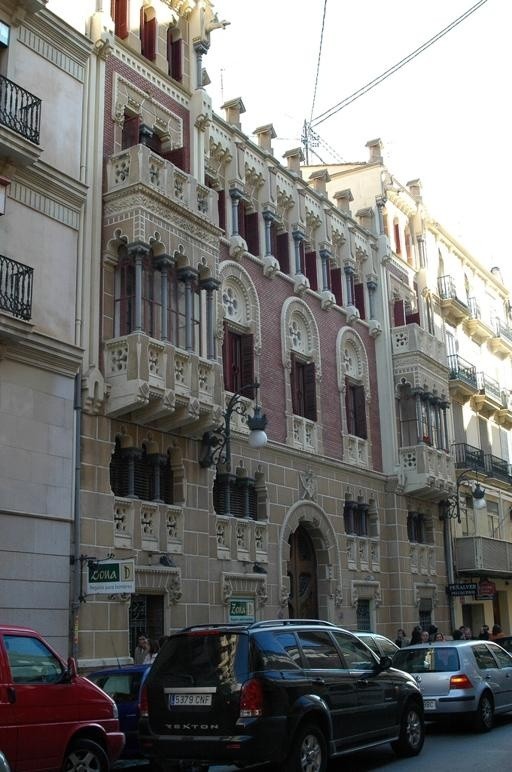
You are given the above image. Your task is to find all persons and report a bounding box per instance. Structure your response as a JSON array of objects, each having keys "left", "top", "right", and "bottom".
[
  {"left": 142, "top": 639, "right": 159, "bottom": 665},
  {"left": 395, "top": 623, "right": 507, "bottom": 649},
  {"left": 133, "top": 630, "right": 151, "bottom": 665}
]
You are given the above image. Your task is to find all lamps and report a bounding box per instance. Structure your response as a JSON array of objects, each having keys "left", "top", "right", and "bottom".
[
  {"left": 199, "top": 376, "right": 267, "bottom": 470},
  {"left": 439, "top": 467, "right": 488, "bottom": 523}
]
